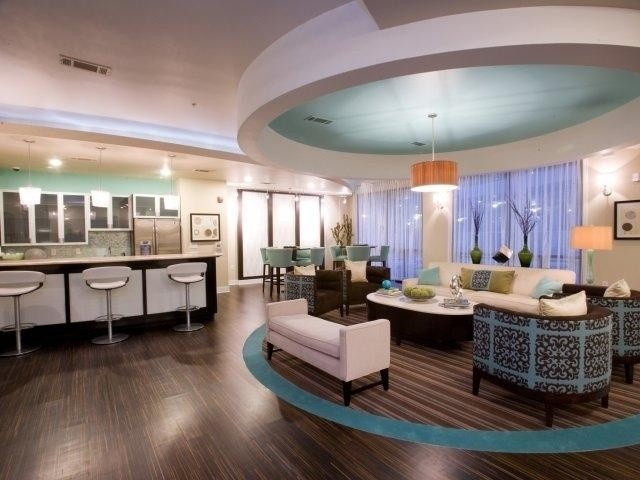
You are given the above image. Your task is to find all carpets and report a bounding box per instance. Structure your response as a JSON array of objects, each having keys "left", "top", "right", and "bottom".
[{"left": 239, "top": 300, "right": 639, "bottom": 455}]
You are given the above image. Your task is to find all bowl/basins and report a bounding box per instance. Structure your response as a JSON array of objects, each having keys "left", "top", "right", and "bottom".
[{"left": 403, "top": 293, "right": 436, "bottom": 303}]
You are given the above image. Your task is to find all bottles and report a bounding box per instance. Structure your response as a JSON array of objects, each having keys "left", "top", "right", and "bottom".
[{"left": 108, "top": 248, "right": 112, "bottom": 257}]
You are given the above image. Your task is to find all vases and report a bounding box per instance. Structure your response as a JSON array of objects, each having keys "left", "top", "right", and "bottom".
[
  {"left": 517, "top": 234, "right": 534, "bottom": 267},
  {"left": 469, "top": 235, "right": 484, "bottom": 265}
]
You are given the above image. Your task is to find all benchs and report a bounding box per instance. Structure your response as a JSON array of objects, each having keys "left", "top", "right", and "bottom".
[{"left": 262, "top": 296, "right": 393, "bottom": 411}]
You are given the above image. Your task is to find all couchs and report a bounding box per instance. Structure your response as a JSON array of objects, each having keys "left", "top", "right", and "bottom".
[{"left": 400, "top": 259, "right": 577, "bottom": 317}]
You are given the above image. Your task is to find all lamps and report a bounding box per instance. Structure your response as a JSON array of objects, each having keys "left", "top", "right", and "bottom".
[
  {"left": 602, "top": 174, "right": 613, "bottom": 197},
  {"left": 163, "top": 154, "right": 182, "bottom": 212},
  {"left": 409, "top": 109, "right": 462, "bottom": 194},
  {"left": 571, "top": 222, "right": 614, "bottom": 286},
  {"left": 89, "top": 143, "right": 111, "bottom": 209},
  {"left": 18, "top": 138, "right": 42, "bottom": 206}
]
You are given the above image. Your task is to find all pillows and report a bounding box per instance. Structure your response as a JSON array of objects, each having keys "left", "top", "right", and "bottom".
[
  {"left": 537, "top": 290, "right": 589, "bottom": 317},
  {"left": 526, "top": 273, "right": 565, "bottom": 301},
  {"left": 601, "top": 275, "right": 632, "bottom": 300},
  {"left": 418, "top": 266, "right": 441, "bottom": 286},
  {"left": 457, "top": 266, "right": 516, "bottom": 294}
]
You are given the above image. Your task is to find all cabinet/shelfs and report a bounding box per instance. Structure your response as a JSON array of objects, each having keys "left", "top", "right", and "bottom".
[
  {"left": 30, "top": 191, "right": 89, "bottom": 247},
  {"left": 86, "top": 193, "right": 134, "bottom": 232},
  {"left": 0, "top": 189, "right": 34, "bottom": 248},
  {"left": 131, "top": 194, "right": 182, "bottom": 219},
  {"left": 128, "top": 217, "right": 181, "bottom": 258}
]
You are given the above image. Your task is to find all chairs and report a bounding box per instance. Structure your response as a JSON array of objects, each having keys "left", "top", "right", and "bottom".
[
  {"left": 164, "top": 261, "right": 211, "bottom": 333},
  {"left": 549, "top": 280, "right": 640, "bottom": 385},
  {"left": 468, "top": 292, "right": 614, "bottom": 427},
  {"left": 257, "top": 241, "right": 393, "bottom": 321},
  {"left": 79, "top": 265, "right": 135, "bottom": 345},
  {"left": 0, "top": 270, "right": 50, "bottom": 360}
]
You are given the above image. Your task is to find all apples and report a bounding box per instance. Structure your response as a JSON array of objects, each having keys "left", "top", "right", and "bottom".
[{"left": 404, "top": 287, "right": 433, "bottom": 296}]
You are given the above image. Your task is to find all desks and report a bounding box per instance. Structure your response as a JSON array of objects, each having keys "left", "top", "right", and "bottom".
[{"left": 0, "top": 249, "right": 224, "bottom": 354}]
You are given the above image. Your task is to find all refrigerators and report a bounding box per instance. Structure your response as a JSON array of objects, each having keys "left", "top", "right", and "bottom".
[{"left": 131, "top": 217, "right": 179, "bottom": 253}]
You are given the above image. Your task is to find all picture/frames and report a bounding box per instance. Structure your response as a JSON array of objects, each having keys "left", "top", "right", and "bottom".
[
  {"left": 612, "top": 199, "right": 640, "bottom": 241},
  {"left": 190, "top": 213, "right": 221, "bottom": 242}
]
